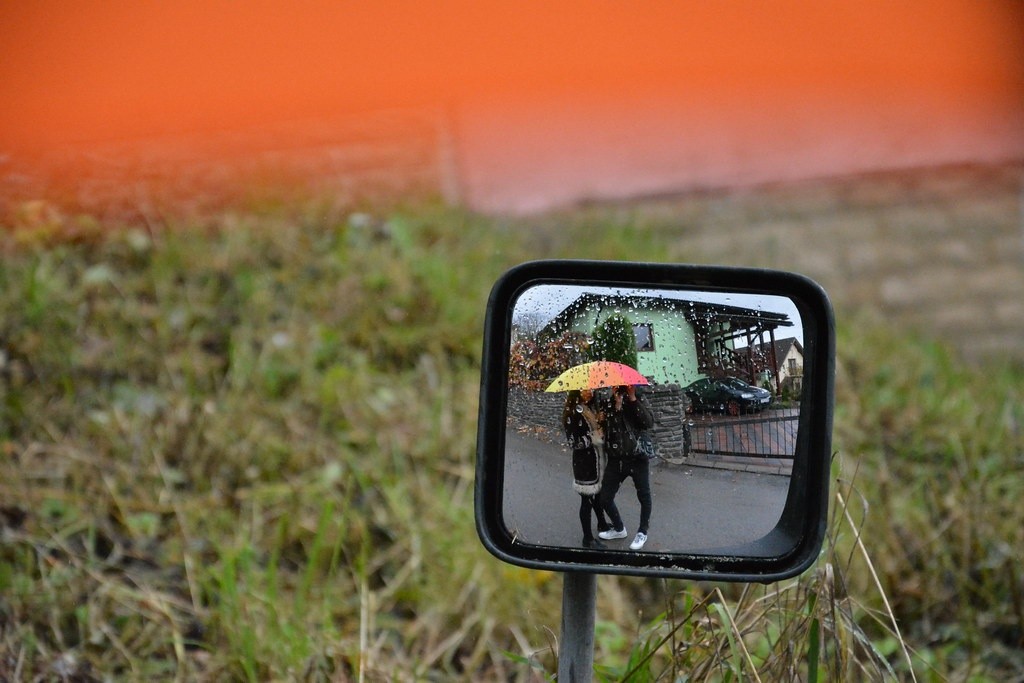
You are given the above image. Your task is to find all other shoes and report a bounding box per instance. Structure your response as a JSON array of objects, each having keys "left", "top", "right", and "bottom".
[
  {"left": 582, "top": 538, "right": 608, "bottom": 549},
  {"left": 597, "top": 523, "right": 614, "bottom": 531}
]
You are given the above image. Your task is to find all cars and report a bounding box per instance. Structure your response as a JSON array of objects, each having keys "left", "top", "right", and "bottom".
[{"left": 682, "top": 376, "right": 772, "bottom": 415}]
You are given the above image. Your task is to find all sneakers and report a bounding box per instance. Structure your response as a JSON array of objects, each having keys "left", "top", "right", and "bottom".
[
  {"left": 599, "top": 527, "right": 628, "bottom": 539},
  {"left": 629, "top": 533, "right": 648, "bottom": 549}
]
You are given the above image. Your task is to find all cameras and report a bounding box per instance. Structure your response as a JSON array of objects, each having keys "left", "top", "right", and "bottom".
[{"left": 616, "top": 385, "right": 628, "bottom": 396}]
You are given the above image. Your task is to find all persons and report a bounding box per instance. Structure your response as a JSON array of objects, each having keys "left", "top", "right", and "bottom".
[
  {"left": 598, "top": 385, "right": 654, "bottom": 549},
  {"left": 563, "top": 390, "right": 612, "bottom": 550}
]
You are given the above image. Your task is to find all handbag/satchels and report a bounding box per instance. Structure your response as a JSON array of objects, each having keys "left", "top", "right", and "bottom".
[{"left": 632, "top": 432, "right": 656, "bottom": 458}]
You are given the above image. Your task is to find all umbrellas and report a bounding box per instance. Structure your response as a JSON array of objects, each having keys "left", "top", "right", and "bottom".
[{"left": 545, "top": 361, "right": 647, "bottom": 411}]
